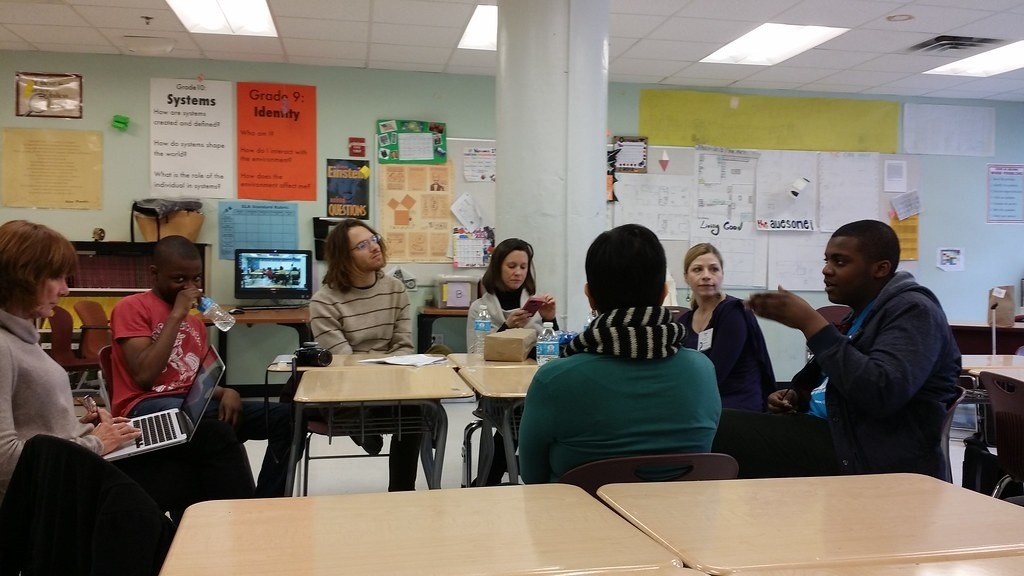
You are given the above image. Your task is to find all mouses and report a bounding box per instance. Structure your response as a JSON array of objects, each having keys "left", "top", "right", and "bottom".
[{"left": 229, "top": 309, "right": 243, "bottom": 314}]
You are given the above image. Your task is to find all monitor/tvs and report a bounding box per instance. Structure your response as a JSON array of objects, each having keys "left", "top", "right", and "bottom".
[{"left": 233, "top": 249, "right": 314, "bottom": 304}]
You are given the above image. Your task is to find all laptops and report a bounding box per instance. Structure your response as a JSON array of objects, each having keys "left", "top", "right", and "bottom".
[{"left": 101, "top": 345, "right": 225, "bottom": 463}]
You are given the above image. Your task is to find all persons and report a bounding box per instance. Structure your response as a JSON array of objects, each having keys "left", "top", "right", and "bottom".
[
  {"left": 308, "top": 218, "right": 425, "bottom": 493},
  {"left": 711, "top": 220, "right": 963, "bottom": 485},
  {"left": 675, "top": 243, "right": 778, "bottom": 414},
  {"left": 111, "top": 235, "right": 308, "bottom": 530},
  {"left": 469, "top": 238, "right": 560, "bottom": 488},
  {"left": 267, "top": 266, "right": 300, "bottom": 283},
  {"left": 0, "top": 220, "right": 143, "bottom": 506},
  {"left": 518, "top": 224, "right": 721, "bottom": 485}
]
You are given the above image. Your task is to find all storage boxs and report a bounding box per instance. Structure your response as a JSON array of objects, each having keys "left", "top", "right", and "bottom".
[{"left": 433, "top": 276, "right": 478, "bottom": 309}]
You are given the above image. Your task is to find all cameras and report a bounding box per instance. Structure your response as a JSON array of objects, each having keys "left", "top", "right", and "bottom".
[{"left": 295, "top": 348, "right": 332, "bottom": 367}]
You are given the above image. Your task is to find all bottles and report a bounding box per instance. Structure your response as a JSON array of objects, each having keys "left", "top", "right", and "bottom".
[
  {"left": 192, "top": 291, "right": 236, "bottom": 332},
  {"left": 583, "top": 309, "right": 594, "bottom": 331},
  {"left": 536, "top": 322, "right": 559, "bottom": 366},
  {"left": 473, "top": 305, "right": 492, "bottom": 360}
]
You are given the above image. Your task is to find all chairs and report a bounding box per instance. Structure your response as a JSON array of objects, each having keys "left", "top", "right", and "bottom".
[
  {"left": 939, "top": 385, "right": 966, "bottom": 484},
  {"left": 74, "top": 300, "right": 111, "bottom": 389},
  {"left": 98, "top": 345, "right": 113, "bottom": 413},
  {"left": 0, "top": 434, "right": 177, "bottom": 576},
  {"left": 559, "top": 453, "right": 739, "bottom": 532},
  {"left": 49, "top": 306, "right": 103, "bottom": 397},
  {"left": 805, "top": 306, "right": 853, "bottom": 363},
  {"left": 980, "top": 371, "right": 1024, "bottom": 499}
]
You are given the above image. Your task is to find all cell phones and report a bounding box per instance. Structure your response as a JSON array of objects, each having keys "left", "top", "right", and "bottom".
[{"left": 522, "top": 297, "right": 544, "bottom": 318}]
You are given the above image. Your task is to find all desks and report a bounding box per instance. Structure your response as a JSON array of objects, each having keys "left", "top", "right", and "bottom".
[{"left": 159, "top": 304, "right": 1024, "bottom": 576}]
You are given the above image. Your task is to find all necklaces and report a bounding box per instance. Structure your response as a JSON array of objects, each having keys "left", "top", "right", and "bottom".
[{"left": 698, "top": 294, "right": 721, "bottom": 333}]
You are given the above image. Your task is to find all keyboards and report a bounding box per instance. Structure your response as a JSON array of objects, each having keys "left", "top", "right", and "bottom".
[{"left": 236, "top": 304, "right": 301, "bottom": 310}]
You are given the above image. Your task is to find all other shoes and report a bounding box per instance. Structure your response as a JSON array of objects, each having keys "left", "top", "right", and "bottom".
[{"left": 350, "top": 432, "right": 382, "bottom": 455}]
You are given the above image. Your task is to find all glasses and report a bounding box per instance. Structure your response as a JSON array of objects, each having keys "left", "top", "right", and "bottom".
[{"left": 349, "top": 233, "right": 381, "bottom": 251}]
